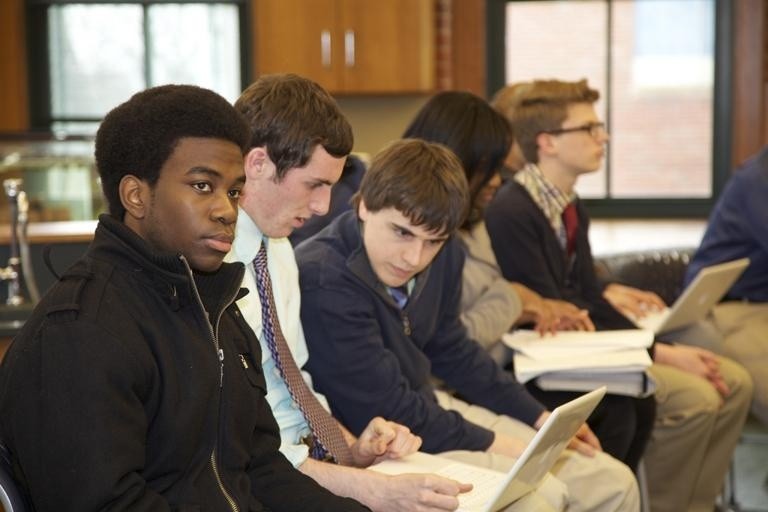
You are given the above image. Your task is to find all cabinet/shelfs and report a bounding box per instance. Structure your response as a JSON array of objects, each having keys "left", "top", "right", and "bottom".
[{"left": 245, "top": 0, "right": 436, "bottom": 98}]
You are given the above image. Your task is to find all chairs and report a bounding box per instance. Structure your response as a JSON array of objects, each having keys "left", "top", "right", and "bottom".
[{"left": 595, "top": 250, "right": 736, "bottom": 510}]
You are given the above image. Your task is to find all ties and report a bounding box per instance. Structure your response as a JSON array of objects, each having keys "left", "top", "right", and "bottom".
[{"left": 253, "top": 241, "right": 355, "bottom": 467}]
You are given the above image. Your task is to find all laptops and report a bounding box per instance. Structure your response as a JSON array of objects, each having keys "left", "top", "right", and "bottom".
[
  {"left": 625, "top": 258, "right": 750, "bottom": 335},
  {"left": 362, "top": 386, "right": 608, "bottom": 510}
]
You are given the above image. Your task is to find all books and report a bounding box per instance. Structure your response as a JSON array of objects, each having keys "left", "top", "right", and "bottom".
[{"left": 501, "top": 326, "right": 659, "bottom": 399}]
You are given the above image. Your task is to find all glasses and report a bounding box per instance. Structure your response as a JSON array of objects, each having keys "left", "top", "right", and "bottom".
[{"left": 547, "top": 122, "right": 605, "bottom": 137}]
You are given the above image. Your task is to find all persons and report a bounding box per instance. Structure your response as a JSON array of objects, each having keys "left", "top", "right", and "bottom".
[
  {"left": 678, "top": 141, "right": 768, "bottom": 496},
  {"left": 402, "top": 88, "right": 656, "bottom": 476},
  {"left": 1, "top": 82, "right": 372, "bottom": 512},
  {"left": 295, "top": 137, "right": 641, "bottom": 512},
  {"left": 489, "top": 83, "right": 534, "bottom": 184},
  {"left": 487, "top": 80, "right": 756, "bottom": 511},
  {"left": 221, "top": 73, "right": 557, "bottom": 512}
]
